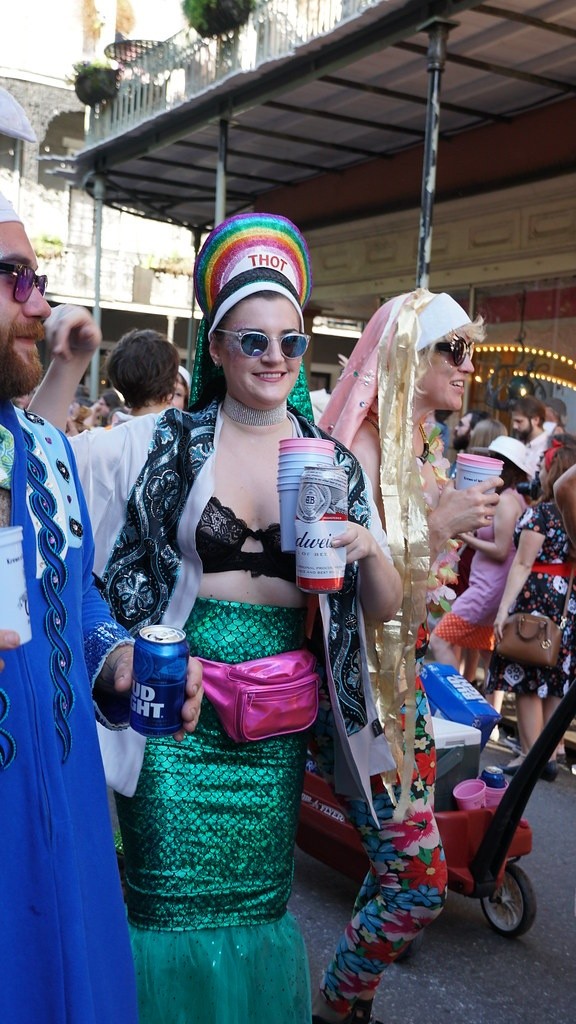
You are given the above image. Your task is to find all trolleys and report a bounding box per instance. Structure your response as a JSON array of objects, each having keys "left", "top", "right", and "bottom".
[{"left": 295, "top": 681, "right": 576, "bottom": 940}]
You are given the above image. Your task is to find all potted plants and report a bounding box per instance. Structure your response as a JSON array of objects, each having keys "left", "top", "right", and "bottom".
[
  {"left": 180, "top": 0, "right": 257, "bottom": 37},
  {"left": 68, "top": 62, "right": 116, "bottom": 106}
]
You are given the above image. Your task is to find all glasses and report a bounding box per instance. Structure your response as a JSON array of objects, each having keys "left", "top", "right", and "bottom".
[
  {"left": 0, "top": 261, "right": 49, "bottom": 304},
  {"left": 424, "top": 334, "right": 476, "bottom": 367},
  {"left": 215, "top": 329, "right": 313, "bottom": 360}
]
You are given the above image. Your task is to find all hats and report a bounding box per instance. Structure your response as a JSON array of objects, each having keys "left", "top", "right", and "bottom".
[
  {"left": 472, "top": 434, "right": 535, "bottom": 479},
  {"left": 0, "top": 191, "right": 24, "bottom": 225}
]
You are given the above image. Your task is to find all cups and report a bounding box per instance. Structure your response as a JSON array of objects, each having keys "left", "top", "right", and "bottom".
[
  {"left": 476, "top": 775, "right": 509, "bottom": 807},
  {"left": 454, "top": 452, "right": 505, "bottom": 519},
  {"left": 276, "top": 437, "right": 337, "bottom": 553},
  {"left": 452, "top": 779, "right": 487, "bottom": 811}
]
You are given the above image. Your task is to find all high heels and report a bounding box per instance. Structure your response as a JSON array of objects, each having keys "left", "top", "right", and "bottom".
[
  {"left": 500, "top": 762, "right": 521, "bottom": 777},
  {"left": 541, "top": 758, "right": 559, "bottom": 782}
]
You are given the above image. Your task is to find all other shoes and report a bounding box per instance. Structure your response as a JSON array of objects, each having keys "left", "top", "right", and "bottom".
[
  {"left": 504, "top": 734, "right": 521, "bottom": 754},
  {"left": 489, "top": 729, "right": 500, "bottom": 742}
]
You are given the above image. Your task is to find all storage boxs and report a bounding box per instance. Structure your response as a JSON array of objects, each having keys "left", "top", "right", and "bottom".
[
  {"left": 418, "top": 662, "right": 501, "bottom": 756},
  {"left": 431, "top": 716, "right": 482, "bottom": 812}
]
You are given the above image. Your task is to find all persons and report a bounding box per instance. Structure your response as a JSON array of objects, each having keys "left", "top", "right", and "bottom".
[
  {"left": 28, "top": 214, "right": 402, "bottom": 1024},
  {"left": 69, "top": 329, "right": 179, "bottom": 577},
  {"left": 11, "top": 365, "right": 193, "bottom": 436},
  {"left": 420, "top": 400, "right": 576, "bottom": 781},
  {"left": 318, "top": 287, "right": 504, "bottom": 1023},
  {"left": 0, "top": 206, "right": 203, "bottom": 1024}
]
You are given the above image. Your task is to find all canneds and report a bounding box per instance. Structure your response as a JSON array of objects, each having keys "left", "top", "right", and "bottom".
[
  {"left": 480, "top": 766, "right": 505, "bottom": 788},
  {"left": 128, "top": 624, "right": 189, "bottom": 737},
  {"left": 295, "top": 465, "right": 349, "bottom": 594}
]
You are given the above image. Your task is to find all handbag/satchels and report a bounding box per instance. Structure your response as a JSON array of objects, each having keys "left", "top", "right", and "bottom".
[
  {"left": 197, "top": 649, "right": 319, "bottom": 743},
  {"left": 496, "top": 611, "right": 562, "bottom": 666}
]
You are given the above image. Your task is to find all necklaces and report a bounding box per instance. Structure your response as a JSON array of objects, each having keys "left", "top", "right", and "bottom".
[
  {"left": 223, "top": 391, "right": 287, "bottom": 425},
  {"left": 416, "top": 422, "right": 429, "bottom": 464}
]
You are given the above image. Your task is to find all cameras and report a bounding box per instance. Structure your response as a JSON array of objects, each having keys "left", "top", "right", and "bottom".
[{"left": 516, "top": 479, "right": 545, "bottom": 500}]
